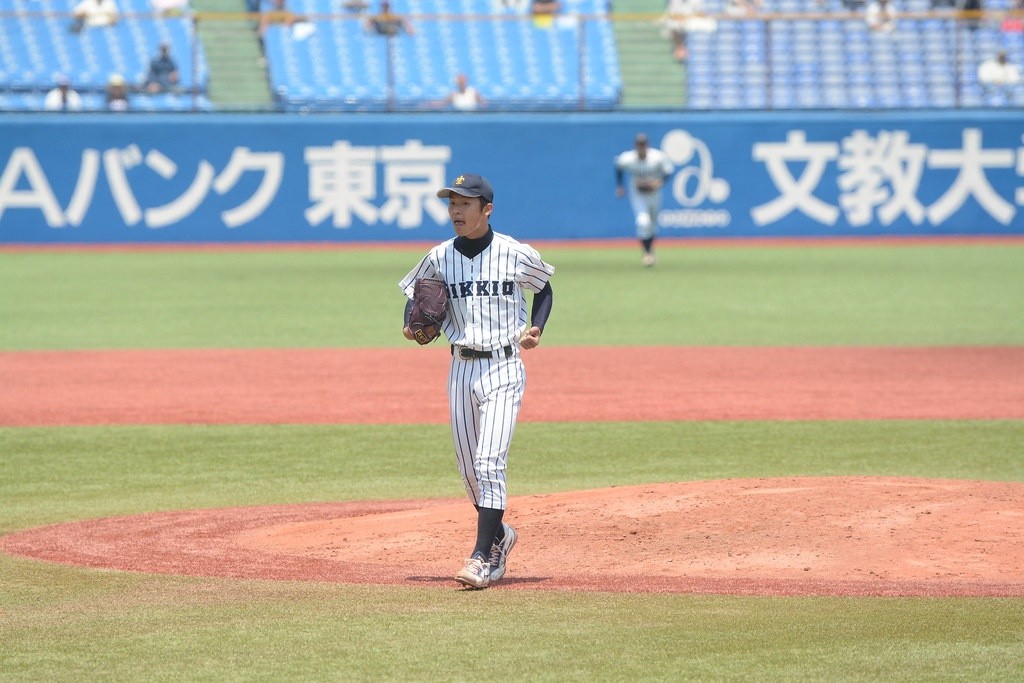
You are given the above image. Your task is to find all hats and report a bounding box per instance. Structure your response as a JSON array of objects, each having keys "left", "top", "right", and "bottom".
[{"left": 437, "top": 174, "right": 493, "bottom": 203}]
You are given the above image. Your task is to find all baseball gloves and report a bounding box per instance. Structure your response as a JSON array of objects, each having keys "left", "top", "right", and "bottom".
[{"left": 408, "top": 276, "right": 449, "bottom": 345}]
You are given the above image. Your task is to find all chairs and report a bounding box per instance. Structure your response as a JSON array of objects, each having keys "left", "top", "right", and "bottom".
[
  {"left": 0, "top": 0, "right": 211, "bottom": 112},
  {"left": 682, "top": 1, "right": 1023, "bottom": 109},
  {"left": 257, "top": 0, "right": 622, "bottom": 111}
]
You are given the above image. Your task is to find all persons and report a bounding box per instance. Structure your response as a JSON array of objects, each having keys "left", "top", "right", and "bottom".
[
  {"left": 429, "top": 71, "right": 484, "bottom": 110},
  {"left": 361, "top": 0, "right": 412, "bottom": 38},
  {"left": 978, "top": 50, "right": 1019, "bottom": 87},
  {"left": 613, "top": 133, "right": 675, "bottom": 264},
  {"left": 399, "top": 175, "right": 557, "bottom": 591},
  {"left": 105, "top": 71, "right": 128, "bottom": 112},
  {"left": 69, "top": 0, "right": 118, "bottom": 31},
  {"left": 866, "top": 2, "right": 896, "bottom": 28},
  {"left": 45, "top": 76, "right": 79, "bottom": 112},
  {"left": 657, "top": 1, "right": 700, "bottom": 61},
  {"left": 141, "top": 44, "right": 178, "bottom": 97},
  {"left": 257, "top": 1, "right": 294, "bottom": 36}
]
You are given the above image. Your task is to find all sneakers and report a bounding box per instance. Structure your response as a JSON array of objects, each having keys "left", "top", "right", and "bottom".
[
  {"left": 454, "top": 558, "right": 490, "bottom": 588},
  {"left": 488, "top": 524, "right": 518, "bottom": 581}
]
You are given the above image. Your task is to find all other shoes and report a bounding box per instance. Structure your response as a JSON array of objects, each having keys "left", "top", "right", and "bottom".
[{"left": 645, "top": 255, "right": 654, "bottom": 263}]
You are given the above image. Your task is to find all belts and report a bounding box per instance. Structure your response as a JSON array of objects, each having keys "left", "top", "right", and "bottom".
[{"left": 451, "top": 345, "right": 513, "bottom": 360}]
